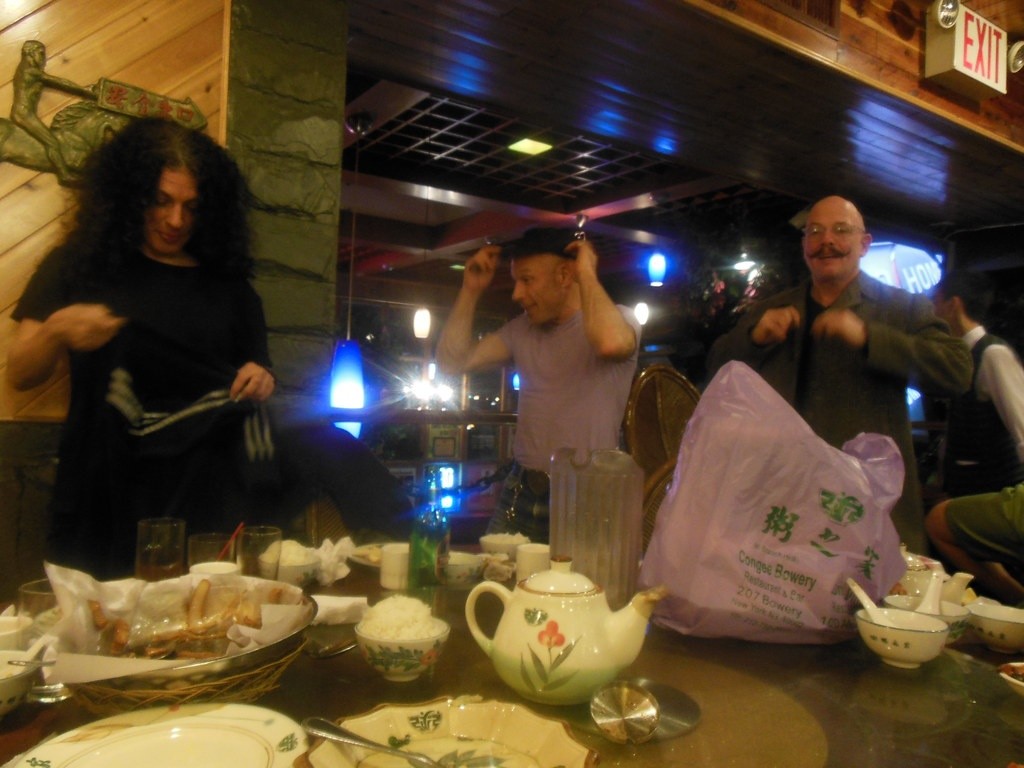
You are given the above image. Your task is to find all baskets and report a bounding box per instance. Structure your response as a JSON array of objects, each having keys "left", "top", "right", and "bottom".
[{"left": 59, "top": 636, "right": 310, "bottom": 717}]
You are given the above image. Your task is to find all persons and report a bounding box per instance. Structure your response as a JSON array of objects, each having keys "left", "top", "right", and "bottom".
[
  {"left": 707, "top": 195, "right": 973, "bottom": 554},
  {"left": 434, "top": 226, "right": 642, "bottom": 544},
  {"left": 6, "top": 117, "right": 278, "bottom": 581},
  {"left": 933, "top": 270, "right": 1024, "bottom": 494},
  {"left": 926, "top": 481, "right": 1024, "bottom": 610}
]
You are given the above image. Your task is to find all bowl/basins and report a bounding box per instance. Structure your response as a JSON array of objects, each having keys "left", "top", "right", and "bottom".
[
  {"left": 449, "top": 561, "right": 485, "bottom": 594},
  {"left": 850, "top": 593, "right": 1022, "bottom": 668},
  {"left": 0, "top": 650, "right": 32, "bottom": 715},
  {"left": 480, "top": 537, "right": 517, "bottom": 562},
  {"left": 258, "top": 556, "right": 321, "bottom": 589},
  {"left": 355, "top": 618, "right": 452, "bottom": 683},
  {"left": 59, "top": 576, "right": 319, "bottom": 718}
]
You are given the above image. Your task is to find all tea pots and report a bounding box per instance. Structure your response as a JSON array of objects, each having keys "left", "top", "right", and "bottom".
[
  {"left": 890, "top": 542, "right": 974, "bottom": 603},
  {"left": 465, "top": 555, "right": 673, "bottom": 705}
]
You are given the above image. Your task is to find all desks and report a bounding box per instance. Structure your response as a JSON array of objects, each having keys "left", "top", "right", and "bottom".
[{"left": 0, "top": 546, "right": 1024, "bottom": 768}]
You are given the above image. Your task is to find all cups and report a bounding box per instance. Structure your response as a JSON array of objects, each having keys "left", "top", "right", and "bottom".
[
  {"left": 0, "top": 614, "right": 33, "bottom": 648},
  {"left": 188, "top": 532, "right": 234, "bottom": 564},
  {"left": 136, "top": 517, "right": 186, "bottom": 579},
  {"left": 237, "top": 527, "right": 282, "bottom": 580},
  {"left": 517, "top": 544, "right": 550, "bottom": 585},
  {"left": 16, "top": 578, "right": 71, "bottom": 702},
  {"left": 188, "top": 562, "right": 237, "bottom": 574},
  {"left": 380, "top": 545, "right": 410, "bottom": 590}
]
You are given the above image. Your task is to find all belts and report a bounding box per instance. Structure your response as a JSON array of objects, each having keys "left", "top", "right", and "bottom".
[{"left": 515, "top": 465, "right": 552, "bottom": 497}]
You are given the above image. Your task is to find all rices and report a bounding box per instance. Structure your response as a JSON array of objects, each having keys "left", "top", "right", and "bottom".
[
  {"left": 357, "top": 595, "right": 442, "bottom": 643},
  {"left": 480, "top": 531, "right": 527, "bottom": 544},
  {"left": 260, "top": 540, "right": 319, "bottom": 567}
]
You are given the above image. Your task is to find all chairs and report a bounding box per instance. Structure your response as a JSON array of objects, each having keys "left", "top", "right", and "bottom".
[
  {"left": 622, "top": 363, "right": 703, "bottom": 464},
  {"left": 640, "top": 457, "right": 676, "bottom": 558}
]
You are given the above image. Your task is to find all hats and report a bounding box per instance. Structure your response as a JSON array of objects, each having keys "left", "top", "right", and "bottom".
[{"left": 494, "top": 226, "right": 577, "bottom": 260}]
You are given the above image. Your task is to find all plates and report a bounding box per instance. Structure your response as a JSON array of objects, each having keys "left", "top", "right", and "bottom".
[
  {"left": 305, "top": 695, "right": 600, "bottom": 768},
  {"left": 348, "top": 543, "right": 386, "bottom": 565},
  {"left": 13, "top": 703, "right": 307, "bottom": 768}
]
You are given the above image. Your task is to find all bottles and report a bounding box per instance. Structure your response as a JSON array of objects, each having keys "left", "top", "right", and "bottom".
[{"left": 409, "top": 468, "right": 451, "bottom": 597}]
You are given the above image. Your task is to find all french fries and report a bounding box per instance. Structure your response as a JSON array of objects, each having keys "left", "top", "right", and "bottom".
[{"left": 188, "top": 580, "right": 212, "bottom": 633}]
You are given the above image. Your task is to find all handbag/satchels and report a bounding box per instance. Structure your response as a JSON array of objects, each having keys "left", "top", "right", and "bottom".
[{"left": 638, "top": 358, "right": 907, "bottom": 645}]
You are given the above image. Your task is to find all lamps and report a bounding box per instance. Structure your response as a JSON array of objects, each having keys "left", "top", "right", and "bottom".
[
  {"left": 734, "top": 246, "right": 755, "bottom": 269},
  {"left": 413, "top": 303, "right": 431, "bottom": 339},
  {"left": 648, "top": 247, "right": 667, "bottom": 287},
  {"left": 923, "top": 0, "right": 1024, "bottom": 97},
  {"left": 330, "top": 121, "right": 366, "bottom": 440}
]
